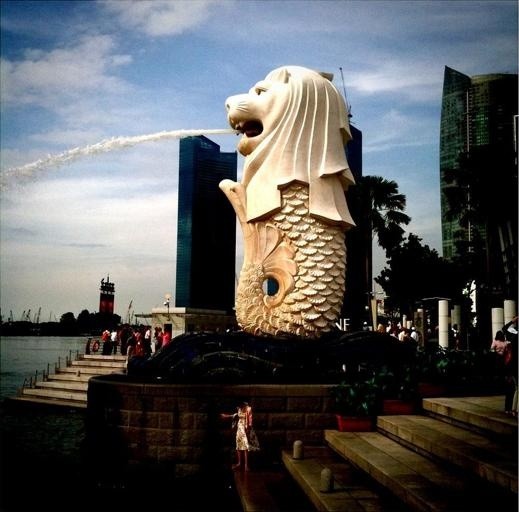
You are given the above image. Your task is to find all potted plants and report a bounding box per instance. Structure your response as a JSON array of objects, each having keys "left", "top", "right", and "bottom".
[{"left": 330, "top": 347, "right": 448, "bottom": 432}]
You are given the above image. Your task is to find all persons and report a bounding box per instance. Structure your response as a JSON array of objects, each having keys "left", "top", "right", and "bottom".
[
  {"left": 499, "top": 315, "right": 519, "bottom": 418},
  {"left": 220, "top": 397, "right": 261, "bottom": 475},
  {"left": 361, "top": 314, "right": 421, "bottom": 346},
  {"left": 489, "top": 330, "right": 513, "bottom": 353},
  {"left": 99, "top": 319, "right": 170, "bottom": 367}
]
